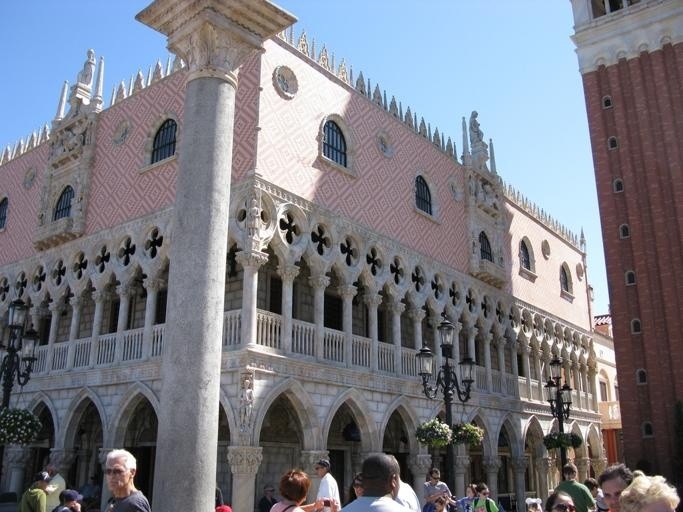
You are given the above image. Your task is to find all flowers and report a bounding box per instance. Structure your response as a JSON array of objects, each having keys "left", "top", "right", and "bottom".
[
  {"left": 0, "top": 406, "right": 43, "bottom": 445},
  {"left": 415, "top": 415, "right": 455, "bottom": 448},
  {"left": 453, "top": 421, "right": 484, "bottom": 447}
]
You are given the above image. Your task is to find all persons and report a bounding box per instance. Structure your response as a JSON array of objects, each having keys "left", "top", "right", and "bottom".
[
  {"left": 76, "top": 48, "right": 96, "bottom": 89},
  {"left": 469, "top": 111, "right": 483, "bottom": 144},
  {"left": 237, "top": 379, "right": 254, "bottom": 432},
  {"left": 20, "top": 464, "right": 100, "bottom": 512},
  {"left": 104, "top": 448, "right": 152, "bottom": 512},
  {"left": 215, "top": 452, "right": 681, "bottom": 512}
]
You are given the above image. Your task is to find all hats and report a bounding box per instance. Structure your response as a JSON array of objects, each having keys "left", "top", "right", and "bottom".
[{"left": 59, "top": 490, "right": 84, "bottom": 501}]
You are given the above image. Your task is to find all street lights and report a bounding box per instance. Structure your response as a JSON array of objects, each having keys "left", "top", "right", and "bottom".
[
  {"left": 544, "top": 354, "right": 572, "bottom": 482},
  {"left": 0, "top": 288, "right": 40, "bottom": 479},
  {"left": 414, "top": 314, "right": 476, "bottom": 512}
]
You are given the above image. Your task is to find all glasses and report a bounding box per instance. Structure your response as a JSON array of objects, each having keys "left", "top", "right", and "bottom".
[{"left": 555, "top": 504, "right": 576, "bottom": 511}]
